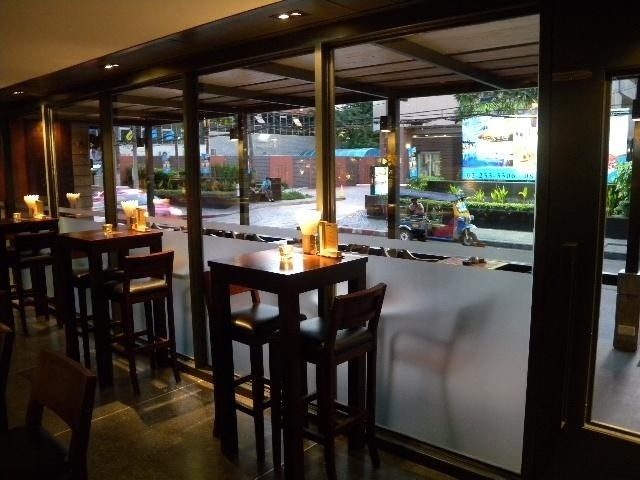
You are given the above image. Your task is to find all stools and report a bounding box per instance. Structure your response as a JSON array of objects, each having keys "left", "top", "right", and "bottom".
[
  {"left": 104, "top": 250, "right": 182, "bottom": 398},
  {"left": 72, "top": 267, "right": 124, "bottom": 369},
  {"left": 268, "top": 282, "right": 387, "bottom": 478},
  {"left": 7, "top": 229, "right": 62, "bottom": 327},
  {"left": 205, "top": 269, "right": 307, "bottom": 465}
]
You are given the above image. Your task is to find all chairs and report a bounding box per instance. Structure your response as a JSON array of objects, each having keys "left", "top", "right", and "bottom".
[
  {"left": 384, "top": 296, "right": 504, "bottom": 451},
  {"left": 382, "top": 246, "right": 439, "bottom": 264}
]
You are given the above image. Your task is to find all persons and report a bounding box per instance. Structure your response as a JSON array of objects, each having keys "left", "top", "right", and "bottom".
[
  {"left": 248, "top": 177, "right": 261, "bottom": 203},
  {"left": 407, "top": 198, "right": 425, "bottom": 218},
  {"left": 258, "top": 176, "right": 275, "bottom": 202}
]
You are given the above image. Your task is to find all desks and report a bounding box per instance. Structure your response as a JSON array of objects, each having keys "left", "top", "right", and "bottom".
[{"left": 434, "top": 257, "right": 509, "bottom": 271}]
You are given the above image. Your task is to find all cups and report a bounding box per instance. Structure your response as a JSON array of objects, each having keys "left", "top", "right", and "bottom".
[{"left": 302, "top": 234, "right": 317, "bottom": 255}]
